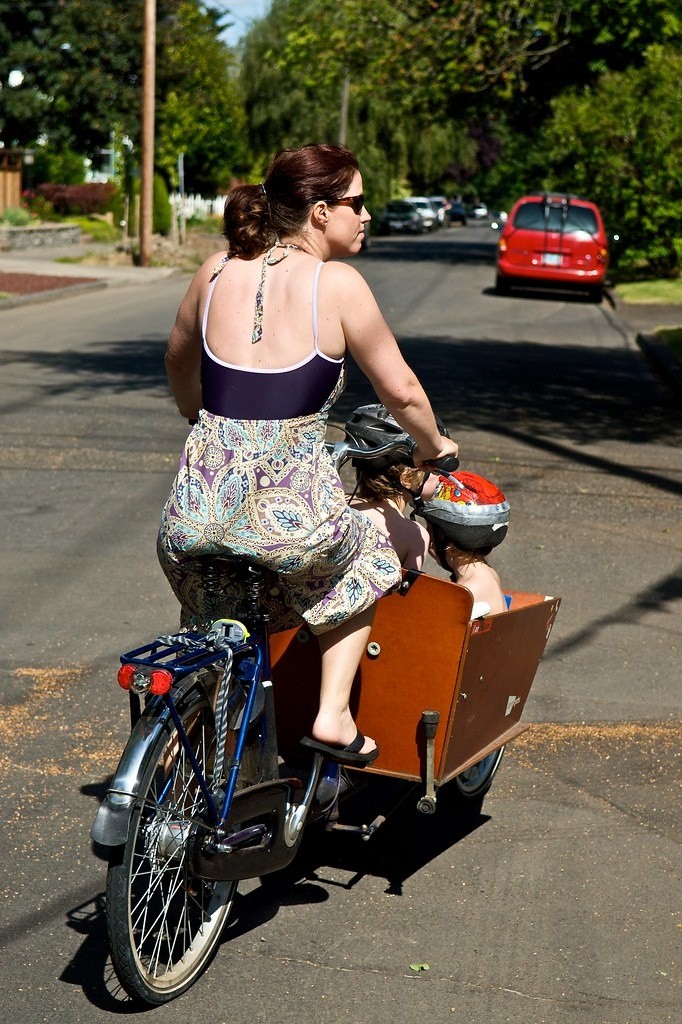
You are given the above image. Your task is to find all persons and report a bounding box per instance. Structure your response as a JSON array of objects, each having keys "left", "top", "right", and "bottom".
[
  {"left": 408, "top": 471, "right": 511, "bottom": 620},
  {"left": 341, "top": 404, "right": 450, "bottom": 572},
  {"left": 156, "top": 144, "right": 460, "bottom": 763}
]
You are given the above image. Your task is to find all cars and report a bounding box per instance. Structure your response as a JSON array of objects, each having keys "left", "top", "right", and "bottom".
[
  {"left": 381, "top": 195, "right": 489, "bottom": 235},
  {"left": 496, "top": 192, "right": 608, "bottom": 303}
]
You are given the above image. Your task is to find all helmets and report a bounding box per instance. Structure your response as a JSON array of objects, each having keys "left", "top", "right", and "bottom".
[
  {"left": 345, "top": 403, "right": 450, "bottom": 463},
  {"left": 410, "top": 471, "right": 510, "bottom": 556}
]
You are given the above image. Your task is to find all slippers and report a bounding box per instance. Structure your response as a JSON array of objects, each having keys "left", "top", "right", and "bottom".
[{"left": 300, "top": 730, "right": 379, "bottom": 760}]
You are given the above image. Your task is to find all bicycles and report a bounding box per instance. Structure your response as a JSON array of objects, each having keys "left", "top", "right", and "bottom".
[{"left": 89, "top": 413, "right": 562, "bottom": 1007}]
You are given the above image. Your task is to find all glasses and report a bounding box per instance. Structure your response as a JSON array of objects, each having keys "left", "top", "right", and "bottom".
[{"left": 310, "top": 194, "right": 365, "bottom": 215}]
